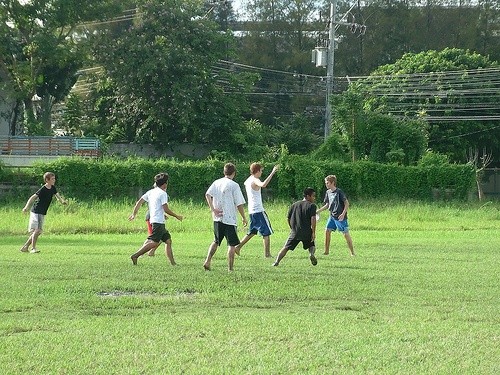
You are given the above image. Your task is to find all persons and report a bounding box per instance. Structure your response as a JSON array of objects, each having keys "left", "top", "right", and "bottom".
[
  {"left": 129, "top": 172, "right": 183, "bottom": 267},
  {"left": 235, "top": 163, "right": 279, "bottom": 257},
  {"left": 273, "top": 187, "right": 317, "bottom": 268},
  {"left": 317, "top": 175, "right": 355, "bottom": 256},
  {"left": 20, "top": 172, "right": 67, "bottom": 253},
  {"left": 204, "top": 163, "right": 248, "bottom": 272}
]
